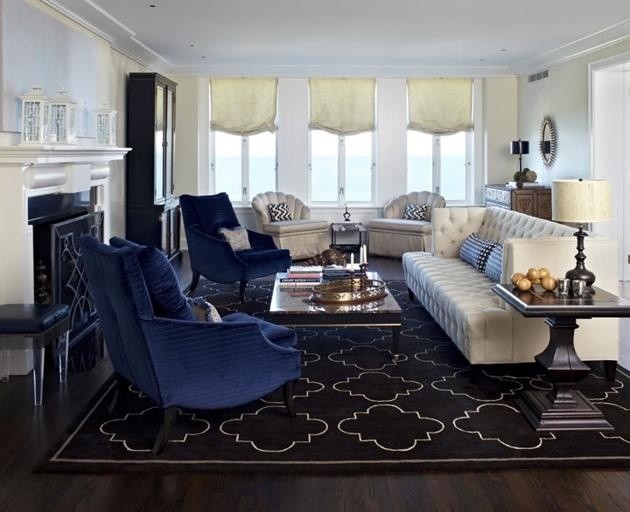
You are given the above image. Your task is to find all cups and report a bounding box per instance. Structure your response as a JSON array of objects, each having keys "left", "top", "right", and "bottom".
[
  {"left": 572, "top": 280, "right": 587, "bottom": 296},
  {"left": 557, "top": 279, "right": 571, "bottom": 294}
]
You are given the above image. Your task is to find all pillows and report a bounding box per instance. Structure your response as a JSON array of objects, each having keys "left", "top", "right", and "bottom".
[
  {"left": 184, "top": 295, "right": 223, "bottom": 325},
  {"left": 107, "top": 234, "right": 191, "bottom": 320},
  {"left": 216, "top": 224, "right": 255, "bottom": 253},
  {"left": 457, "top": 229, "right": 498, "bottom": 274},
  {"left": 267, "top": 202, "right": 293, "bottom": 223},
  {"left": 484, "top": 242, "right": 505, "bottom": 284},
  {"left": 402, "top": 200, "right": 430, "bottom": 222}
]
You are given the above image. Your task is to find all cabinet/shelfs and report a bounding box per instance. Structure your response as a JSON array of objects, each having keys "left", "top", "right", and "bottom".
[
  {"left": 123, "top": 71, "right": 184, "bottom": 269},
  {"left": 484, "top": 180, "right": 564, "bottom": 225}
]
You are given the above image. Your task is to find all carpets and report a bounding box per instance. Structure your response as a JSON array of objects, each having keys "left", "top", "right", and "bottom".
[{"left": 25, "top": 277, "right": 629, "bottom": 481}]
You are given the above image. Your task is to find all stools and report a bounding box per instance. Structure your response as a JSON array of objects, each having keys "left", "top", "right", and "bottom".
[{"left": 1, "top": 301, "right": 76, "bottom": 410}]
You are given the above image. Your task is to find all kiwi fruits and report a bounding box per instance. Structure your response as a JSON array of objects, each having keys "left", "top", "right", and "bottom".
[{"left": 511, "top": 268, "right": 557, "bottom": 291}]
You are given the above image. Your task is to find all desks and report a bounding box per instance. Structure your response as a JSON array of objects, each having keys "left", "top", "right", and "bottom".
[
  {"left": 330, "top": 219, "right": 367, "bottom": 251},
  {"left": 489, "top": 277, "right": 630, "bottom": 434}
]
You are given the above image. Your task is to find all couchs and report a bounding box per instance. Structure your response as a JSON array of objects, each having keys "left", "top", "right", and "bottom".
[
  {"left": 173, "top": 191, "right": 296, "bottom": 301},
  {"left": 73, "top": 234, "right": 309, "bottom": 456},
  {"left": 248, "top": 188, "right": 334, "bottom": 261},
  {"left": 396, "top": 204, "right": 624, "bottom": 386},
  {"left": 365, "top": 189, "right": 448, "bottom": 258}
]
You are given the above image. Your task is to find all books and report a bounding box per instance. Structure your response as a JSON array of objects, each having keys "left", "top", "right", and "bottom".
[{"left": 278, "top": 264, "right": 322, "bottom": 289}]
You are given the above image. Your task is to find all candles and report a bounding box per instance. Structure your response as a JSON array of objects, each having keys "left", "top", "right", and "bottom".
[
  {"left": 349, "top": 251, "right": 355, "bottom": 273},
  {"left": 359, "top": 246, "right": 364, "bottom": 266},
  {"left": 362, "top": 243, "right": 368, "bottom": 264}
]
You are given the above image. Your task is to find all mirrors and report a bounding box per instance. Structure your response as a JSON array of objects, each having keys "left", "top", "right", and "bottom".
[{"left": 538, "top": 115, "right": 559, "bottom": 168}]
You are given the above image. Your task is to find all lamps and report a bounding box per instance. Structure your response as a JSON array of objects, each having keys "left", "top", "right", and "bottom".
[
  {"left": 549, "top": 176, "right": 618, "bottom": 297},
  {"left": 510, "top": 137, "right": 530, "bottom": 174}
]
